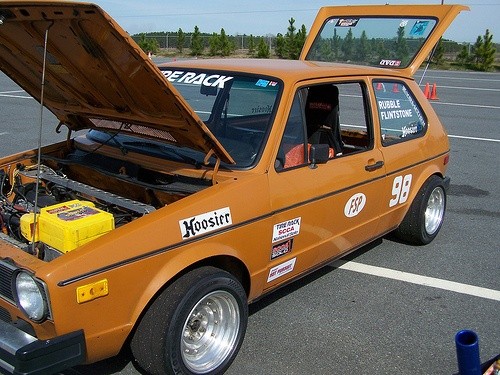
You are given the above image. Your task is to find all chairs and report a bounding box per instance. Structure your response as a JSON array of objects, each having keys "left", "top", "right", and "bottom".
[{"left": 294, "top": 84, "right": 343, "bottom": 156}]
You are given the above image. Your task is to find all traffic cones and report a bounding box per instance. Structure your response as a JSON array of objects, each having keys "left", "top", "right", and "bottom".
[
  {"left": 390, "top": 83, "right": 400, "bottom": 92},
  {"left": 429, "top": 82, "right": 439, "bottom": 100},
  {"left": 376, "top": 82, "right": 383, "bottom": 90},
  {"left": 423, "top": 82, "right": 430, "bottom": 99}
]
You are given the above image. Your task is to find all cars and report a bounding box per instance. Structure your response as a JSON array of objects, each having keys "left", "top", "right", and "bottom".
[{"left": 0, "top": 1, "right": 471, "bottom": 374}]
